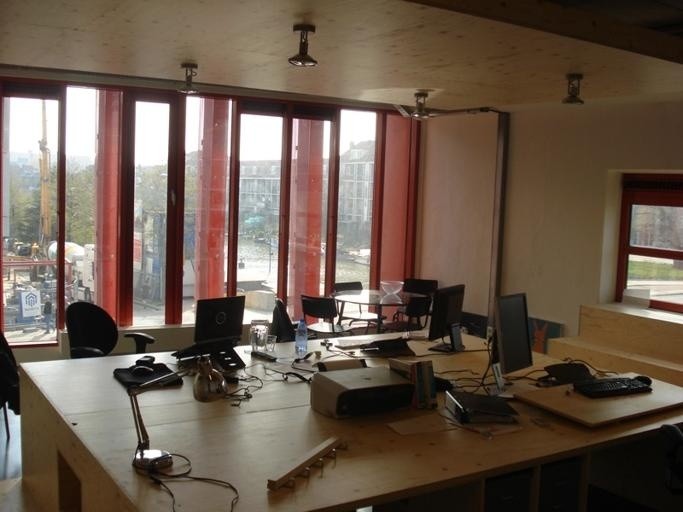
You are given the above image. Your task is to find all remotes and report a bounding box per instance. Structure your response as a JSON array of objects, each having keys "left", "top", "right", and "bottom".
[{"left": 251, "top": 351, "right": 277, "bottom": 362}]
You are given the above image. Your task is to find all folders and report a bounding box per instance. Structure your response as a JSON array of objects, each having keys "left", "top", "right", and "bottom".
[{"left": 445, "top": 388, "right": 520, "bottom": 426}]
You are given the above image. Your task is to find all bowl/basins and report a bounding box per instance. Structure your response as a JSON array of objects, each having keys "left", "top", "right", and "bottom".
[{"left": 380, "top": 281, "right": 404, "bottom": 295}]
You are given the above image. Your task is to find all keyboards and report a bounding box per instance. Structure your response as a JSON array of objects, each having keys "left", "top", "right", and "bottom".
[{"left": 573, "top": 377, "right": 652, "bottom": 399}]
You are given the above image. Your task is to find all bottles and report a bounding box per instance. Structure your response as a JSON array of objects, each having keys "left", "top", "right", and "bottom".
[{"left": 295, "top": 320, "right": 308, "bottom": 356}]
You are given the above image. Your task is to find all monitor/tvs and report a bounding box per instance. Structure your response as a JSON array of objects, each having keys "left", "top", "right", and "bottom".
[
  {"left": 428, "top": 284, "right": 466, "bottom": 352},
  {"left": 489, "top": 292, "right": 540, "bottom": 398}
]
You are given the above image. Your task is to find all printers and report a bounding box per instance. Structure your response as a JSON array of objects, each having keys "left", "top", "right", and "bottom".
[{"left": 310, "top": 358, "right": 413, "bottom": 420}]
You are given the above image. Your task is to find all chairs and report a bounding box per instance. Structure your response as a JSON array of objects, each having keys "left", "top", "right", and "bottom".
[
  {"left": 0, "top": 333, "right": 28, "bottom": 444},
  {"left": 268, "top": 297, "right": 316, "bottom": 341},
  {"left": 334, "top": 281, "right": 361, "bottom": 322},
  {"left": 403, "top": 277, "right": 436, "bottom": 325},
  {"left": 301, "top": 296, "right": 337, "bottom": 337},
  {"left": 375, "top": 292, "right": 433, "bottom": 332},
  {"left": 67, "top": 301, "right": 155, "bottom": 356}
]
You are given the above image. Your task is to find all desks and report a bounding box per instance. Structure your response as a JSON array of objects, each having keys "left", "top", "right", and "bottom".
[
  {"left": 331, "top": 289, "right": 429, "bottom": 334},
  {"left": 21, "top": 312, "right": 682, "bottom": 512}
]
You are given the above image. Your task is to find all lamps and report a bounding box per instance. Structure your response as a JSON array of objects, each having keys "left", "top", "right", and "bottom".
[
  {"left": 562, "top": 73, "right": 587, "bottom": 104},
  {"left": 288, "top": 25, "right": 319, "bottom": 66},
  {"left": 127, "top": 350, "right": 232, "bottom": 473},
  {"left": 411, "top": 92, "right": 429, "bottom": 120},
  {"left": 178, "top": 62, "right": 199, "bottom": 94}
]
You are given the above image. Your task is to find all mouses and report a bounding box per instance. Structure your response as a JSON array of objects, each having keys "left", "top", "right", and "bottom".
[
  {"left": 132, "top": 366, "right": 153, "bottom": 377},
  {"left": 634, "top": 375, "right": 652, "bottom": 386}
]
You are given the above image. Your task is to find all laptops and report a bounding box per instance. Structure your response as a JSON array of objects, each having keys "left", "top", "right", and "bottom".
[{"left": 170, "top": 295, "right": 246, "bottom": 357}]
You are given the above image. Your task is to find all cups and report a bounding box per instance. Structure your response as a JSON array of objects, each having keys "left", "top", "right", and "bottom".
[
  {"left": 250, "top": 320, "right": 269, "bottom": 353},
  {"left": 265, "top": 335, "right": 277, "bottom": 352}
]
